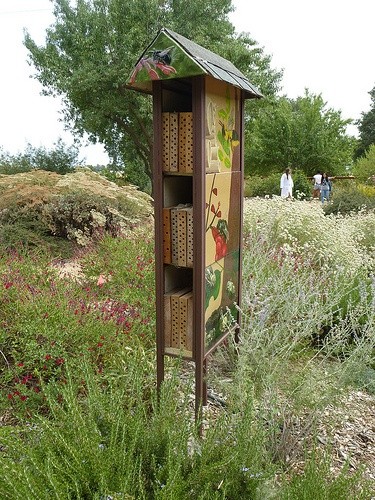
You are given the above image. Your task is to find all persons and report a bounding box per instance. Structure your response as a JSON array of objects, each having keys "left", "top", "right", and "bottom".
[
  {"left": 279, "top": 168, "right": 294, "bottom": 199},
  {"left": 312, "top": 171, "right": 321, "bottom": 198},
  {"left": 319, "top": 172, "right": 334, "bottom": 202}
]
